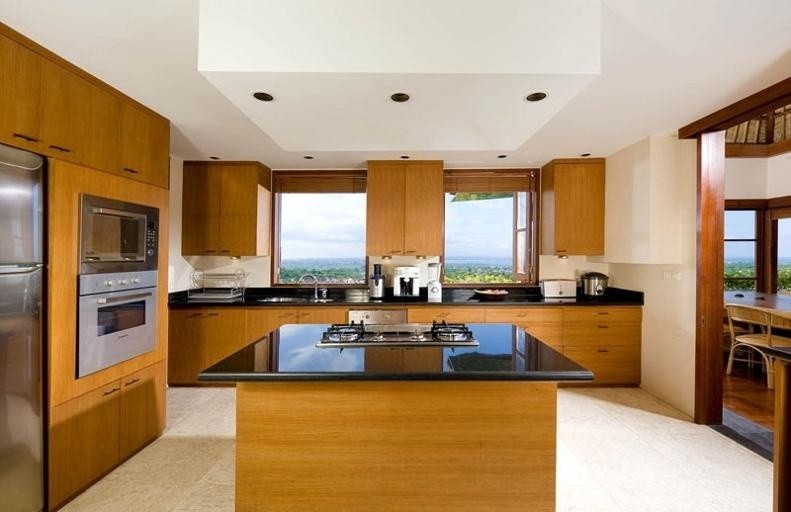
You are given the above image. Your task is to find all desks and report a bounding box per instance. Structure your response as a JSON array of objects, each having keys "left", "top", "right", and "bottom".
[{"left": 196, "top": 321, "right": 597, "bottom": 512}]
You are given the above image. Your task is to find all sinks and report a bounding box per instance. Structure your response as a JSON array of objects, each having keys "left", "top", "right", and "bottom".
[{"left": 257, "top": 296, "right": 306, "bottom": 304}]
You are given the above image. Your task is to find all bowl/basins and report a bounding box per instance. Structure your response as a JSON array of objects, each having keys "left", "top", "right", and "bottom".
[{"left": 345, "top": 288, "right": 371, "bottom": 304}]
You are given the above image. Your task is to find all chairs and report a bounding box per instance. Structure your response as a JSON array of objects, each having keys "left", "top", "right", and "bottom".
[{"left": 723, "top": 289, "right": 790, "bottom": 390}]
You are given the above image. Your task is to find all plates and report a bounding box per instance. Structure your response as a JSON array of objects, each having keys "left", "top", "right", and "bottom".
[{"left": 473, "top": 288, "right": 509, "bottom": 297}]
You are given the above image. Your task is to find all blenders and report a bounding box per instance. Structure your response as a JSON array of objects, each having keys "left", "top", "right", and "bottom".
[{"left": 426, "top": 262, "right": 445, "bottom": 301}]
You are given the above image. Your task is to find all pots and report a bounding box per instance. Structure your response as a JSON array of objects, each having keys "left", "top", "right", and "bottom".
[{"left": 581, "top": 271, "right": 610, "bottom": 299}]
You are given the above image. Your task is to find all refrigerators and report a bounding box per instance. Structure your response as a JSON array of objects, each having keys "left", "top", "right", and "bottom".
[{"left": 0, "top": 143, "right": 45, "bottom": 511}]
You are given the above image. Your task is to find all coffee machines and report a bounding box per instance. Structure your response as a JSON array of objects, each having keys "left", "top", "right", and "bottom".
[{"left": 395, "top": 265, "right": 420, "bottom": 298}]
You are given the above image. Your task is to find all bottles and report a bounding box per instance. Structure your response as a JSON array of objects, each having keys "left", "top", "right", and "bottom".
[{"left": 370, "top": 263, "right": 386, "bottom": 300}]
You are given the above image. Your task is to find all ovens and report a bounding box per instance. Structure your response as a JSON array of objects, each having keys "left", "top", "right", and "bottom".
[{"left": 78, "top": 189, "right": 161, "bottom": 380}]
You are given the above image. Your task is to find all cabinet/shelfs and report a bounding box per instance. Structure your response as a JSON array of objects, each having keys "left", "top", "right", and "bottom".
[
  {"left": 167, "top": 307, "right": 247, "bottom": 388},
  {"left": 181, "top": 159, "right": 272, "bottom": 256},
  {"left": 365, "top": 160, "right": 445, "bottom": 258},
  {"left": 540, "top": 156, "right": 605, "bottom": 256},
  {"left": 247, "top": 307, "right": 349, "bottom": 349},
  {"left": 408, "top": 306, "right": 487, "bottom": 323},
  {"left": 564, "top": 306, "right": 641, "bottom": 388},
  {"left": 47, "top": 356, "right": 168, "bottom": 512},
  {"left": 0, "top": 18, "right": 124, "bottom": 179},
  {"left": 185, "top": 266, "right": 247, "bottom": 305},
  {"left": 487, "top": 306, "right": 564, "bottom": 389},
  {"left": 124, "top": 92, "right": 170, "bottom": 191}
]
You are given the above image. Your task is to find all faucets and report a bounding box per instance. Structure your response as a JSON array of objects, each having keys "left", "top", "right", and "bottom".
[{"left": 297, "top": 273, "right": 319, "bottom": 300}]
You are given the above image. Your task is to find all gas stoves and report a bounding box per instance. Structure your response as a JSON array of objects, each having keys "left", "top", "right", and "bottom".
[{"left": 317, "top": 319, "right": 481, "bottom": 348}]
